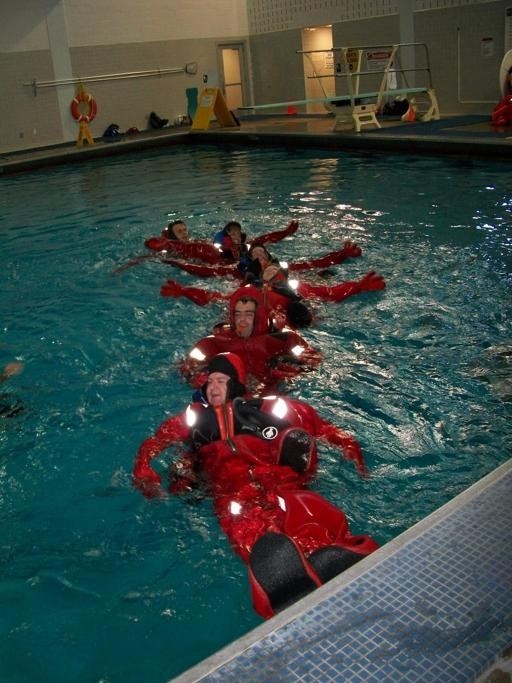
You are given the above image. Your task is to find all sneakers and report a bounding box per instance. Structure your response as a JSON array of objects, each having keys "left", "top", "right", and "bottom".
[
  {"left": 249, "top": 532, "right": 316, "bottom": 616},
  {"left": 276, "top": 429, "right": 313, "bottom": 476},
  {"left": 308, "top": 545, "right": 367, "bottom": 584}
]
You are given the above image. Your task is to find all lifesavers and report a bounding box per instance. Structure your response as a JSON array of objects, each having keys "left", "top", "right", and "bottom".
[{"left": 71, "top": 92, "right": 96, "bottom": 123}]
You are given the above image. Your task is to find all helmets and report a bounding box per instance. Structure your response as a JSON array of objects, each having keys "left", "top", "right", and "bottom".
[
  {"left": 200, "top": 352, "right": 246, "bottom": 402},
  {"left": 230, "top": 286, "right": 268, "bottom": 337}
]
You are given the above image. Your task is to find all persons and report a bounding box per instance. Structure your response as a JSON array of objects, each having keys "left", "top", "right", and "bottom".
[
  {"left": 129, "top": 349, "right": 379, "bottom": 624},
  {"left": 0, "top": 358, "right": 27, "bottom": 421},
  {"left": 142, "top": 218, "right": 302, "bottom": 261},
  {"left": 159, "top": 264, "right": 389, "bottom": 324},
  {"left": 178, "top": 286, "right": 324, "bottom": 396},
  {"left": 152, "top": 240, "right": 362, "bottom": 278},
  {"left": 110, "top": 219, "right": 189, "bottom": 276}
]
